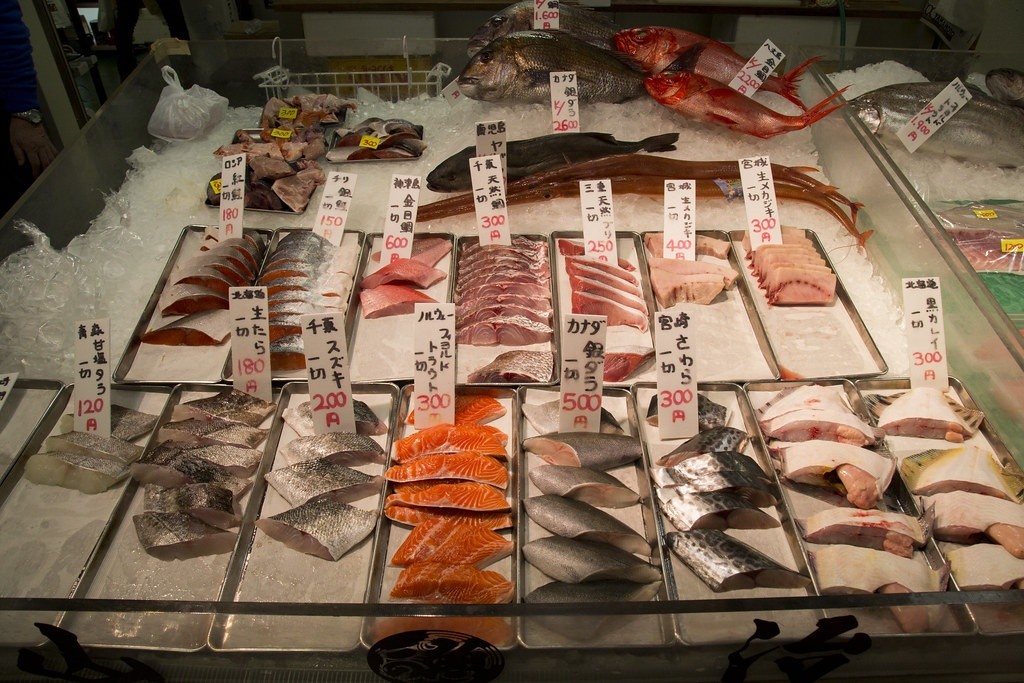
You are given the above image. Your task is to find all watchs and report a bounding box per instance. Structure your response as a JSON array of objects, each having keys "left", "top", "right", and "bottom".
[{"left": 12, "top": 108, "right": 43, "bottom": 125}]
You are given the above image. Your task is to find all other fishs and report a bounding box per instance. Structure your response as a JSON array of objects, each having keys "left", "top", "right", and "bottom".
[{"left": 414, "top": 1, "right": 1024, "bottom": 247}]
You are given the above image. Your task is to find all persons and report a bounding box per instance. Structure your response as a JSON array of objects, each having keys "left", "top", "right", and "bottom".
[{"left": 0, "top": 0, "right": 60, "bottom": 214}]
